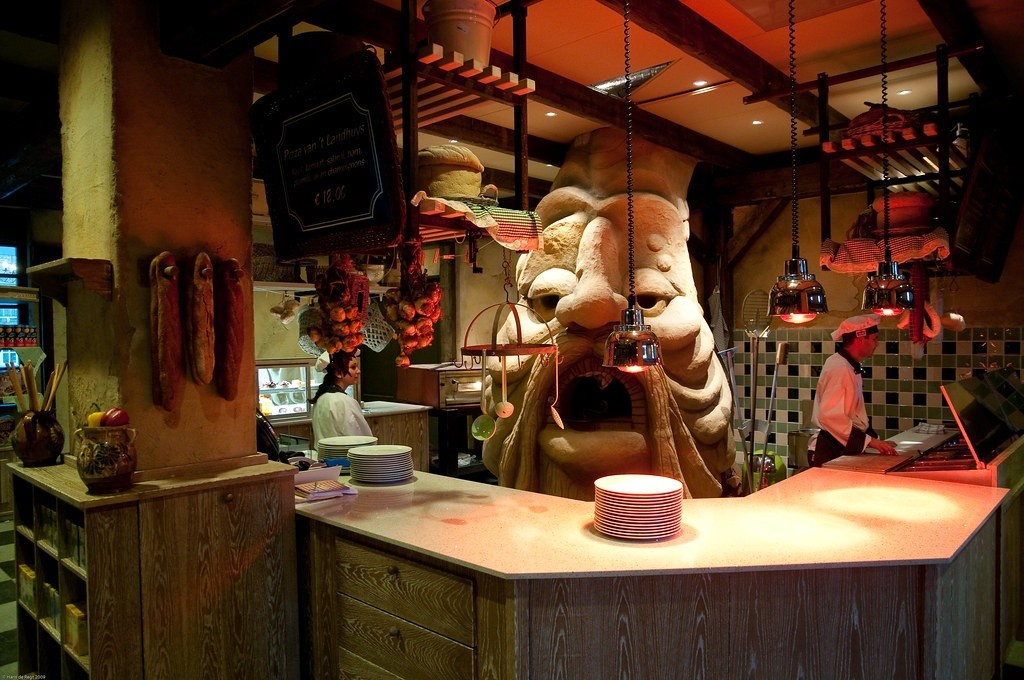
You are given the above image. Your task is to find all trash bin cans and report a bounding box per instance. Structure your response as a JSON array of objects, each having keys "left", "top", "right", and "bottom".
[{"left": 741, "top": 449, "right": 787, "bottom": 493}]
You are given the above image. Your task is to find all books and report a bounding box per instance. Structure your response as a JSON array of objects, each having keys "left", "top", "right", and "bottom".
[{"left": 293, "top": 465, "right": 358, "bottom": 502}]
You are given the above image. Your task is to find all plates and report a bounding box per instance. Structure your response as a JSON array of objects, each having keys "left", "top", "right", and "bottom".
[{"left": 593, "top": 474, "right": 682, "bottom": 539}]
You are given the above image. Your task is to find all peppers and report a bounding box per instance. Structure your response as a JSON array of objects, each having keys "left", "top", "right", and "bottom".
[
  {"left": 86, "top": 403, "right": 106, "bottom": 427},
  {"left": 100, "top": 409, "right": 128, "bottom": 426}
]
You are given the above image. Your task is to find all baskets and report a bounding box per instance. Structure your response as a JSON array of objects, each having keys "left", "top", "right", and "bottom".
[
  {"left": 253, "top": 242, "right": 318, "bottom": 282},
  {"left": 848, "top": 113, "right": 926, "bottom": 136},
  {"left": 360, "top": 302, "right": 395, "bottom": 353},
  {"left": 438, "top": 184, "right": 499, "bottom": 207},
  {"left": 298, "top": 303, "right": 327, "bottom": 357}
]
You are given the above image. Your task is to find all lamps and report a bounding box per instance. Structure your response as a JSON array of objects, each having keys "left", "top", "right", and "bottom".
[
  {"left": 860, "top": 0, "right": 917, "bottom": 320},
  {"left": 603, "top": 0, "right": 662, "bottom": 374},
  {"left": 764, "top": 0, "right": 831, "bottom": 326}
]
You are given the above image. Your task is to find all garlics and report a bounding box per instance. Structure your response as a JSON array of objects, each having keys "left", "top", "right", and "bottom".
[
  {"left": 384, "top": 283, "right": 443, "bottom": 368},
  {"left": 310, "top": 307, "right": 364, "bottom": 353}
]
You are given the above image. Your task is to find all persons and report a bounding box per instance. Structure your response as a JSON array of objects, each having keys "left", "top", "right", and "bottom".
[
  {"left": 812, "top": 314, "right": 899, "bottom": 467},
  {"left": 307, "top": 350, "right": 372, "bottom": 451}
]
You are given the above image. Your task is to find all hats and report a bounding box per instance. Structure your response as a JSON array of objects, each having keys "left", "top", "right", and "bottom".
[
  {"left": 831, "top": 314, "right": 880, "bottom": 341},
  {"left": 315, "top": 348, "right": 360, "bottom": 373}
]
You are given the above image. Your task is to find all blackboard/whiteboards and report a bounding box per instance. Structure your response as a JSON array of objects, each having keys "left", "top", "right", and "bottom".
[{"left": 248, "top": 50, "right": 405, "bottom": 264}]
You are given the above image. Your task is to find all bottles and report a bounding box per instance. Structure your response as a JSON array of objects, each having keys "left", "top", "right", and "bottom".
[
  {"left": 261, "top": 395, "right": 272, "bottom": 412},
  {"left": 317, "top": 436, "right": 413, "bottom": 483}
]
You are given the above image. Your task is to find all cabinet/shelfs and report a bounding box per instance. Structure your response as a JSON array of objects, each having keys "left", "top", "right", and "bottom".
[{"left": 7, "top": 461, "right": 143, "bottom": 680}]
